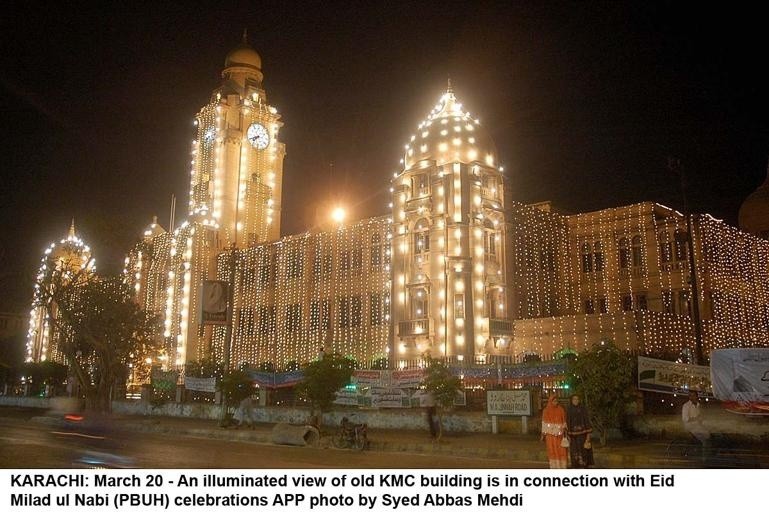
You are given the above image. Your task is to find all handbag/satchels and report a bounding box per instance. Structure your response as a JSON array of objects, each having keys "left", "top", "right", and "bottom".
[
  {"left": 561, "top": 438, "right": 569, "bottom": 448},
  {"left": 584, "top": 440, "right": 592, "bottom": 449}
]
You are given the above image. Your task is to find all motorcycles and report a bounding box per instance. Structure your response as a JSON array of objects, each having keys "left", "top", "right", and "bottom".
[{"left": 332, "top": 415, "right": 368, "bottom": 451}]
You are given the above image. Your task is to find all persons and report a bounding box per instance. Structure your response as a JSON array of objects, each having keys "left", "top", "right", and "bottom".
[
  {"left": 682, "top": 390, "right": 703, "bottom": 457},
  {"left": 540, "top": 394, "right": 568, "bottom": 469},
  {"left": 568, "top": 395, "right": 595, "bottom": 468}
]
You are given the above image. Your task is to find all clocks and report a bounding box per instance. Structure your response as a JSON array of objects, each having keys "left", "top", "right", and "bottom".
[{"left": 245, "top": 122, "right": 271, "bottom": 152}]
